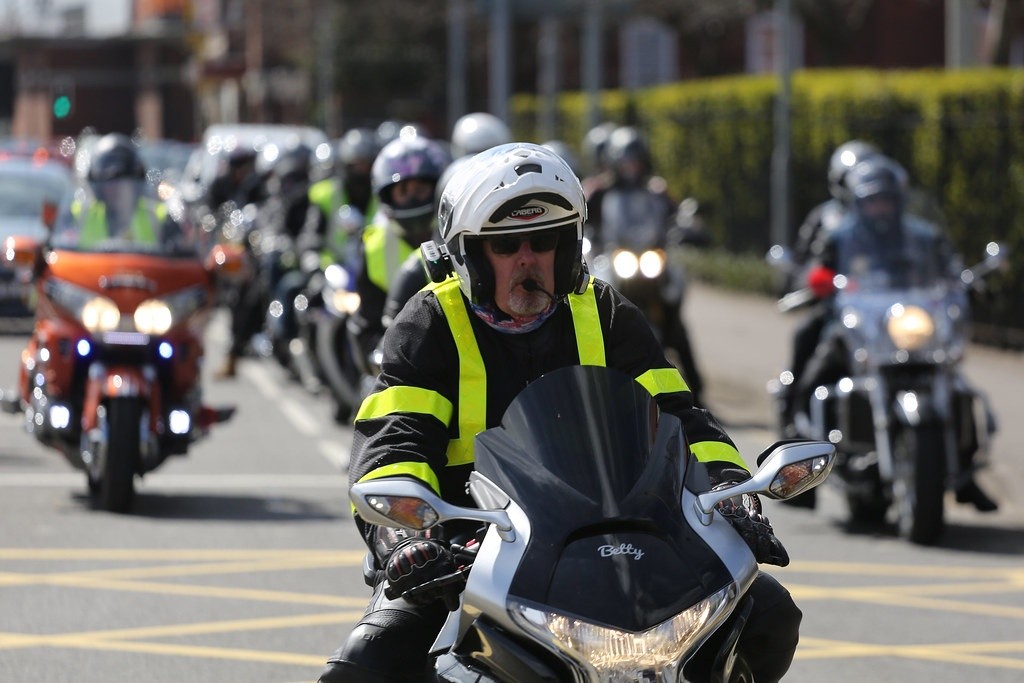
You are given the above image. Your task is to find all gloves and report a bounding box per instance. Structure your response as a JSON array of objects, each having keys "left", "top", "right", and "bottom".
[
  {"left": 387, "top": 538, "right": 467, "bottom": 613},
  {"left": 714, "top": 480, "right": 790, "bottom": 568},
  {"left": 811, "top": 267, "right": 836, "bottom": 296}
]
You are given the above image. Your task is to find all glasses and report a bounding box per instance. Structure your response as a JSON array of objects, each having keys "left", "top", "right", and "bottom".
[{"left": 489, "top": 233, "right": 556, "bottom": 255}]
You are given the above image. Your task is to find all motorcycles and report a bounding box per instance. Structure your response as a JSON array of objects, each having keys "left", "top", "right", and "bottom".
[
  {"left": 7, "top": 232, "right": 250, "bottom": 513},
  {"left": 765, "top": 235, "right": 1008, "bottom": 545},
  {"left": 178, "top": 190, "right": 701, "bottom": 432},
  {"left": 348, "top": 364, "right": 835, "bottom": 683}
]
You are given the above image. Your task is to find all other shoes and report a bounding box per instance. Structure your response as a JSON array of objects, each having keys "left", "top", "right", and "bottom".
[{"left": 955, "top": 483, "right": 998, "bottom": 511}]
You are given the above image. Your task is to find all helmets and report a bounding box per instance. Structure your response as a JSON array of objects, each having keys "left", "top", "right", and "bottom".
[
  {"left": 82, "top": 132, "right": 147, "bottom": 182},
  {"left": 436, "top": 142, "right": 589, "bottom": 305},
  {"left": 583, "top": 121, "right": 654, "bottom": 173},
  {"left": 825, "top": 136, "right": 907, "bottom": 208},
  {"left": 337, "top": 128, "right": 379, "bottom": 165},
  {"left": 370, "top": 136, "right": 453, "bottom": 220}
]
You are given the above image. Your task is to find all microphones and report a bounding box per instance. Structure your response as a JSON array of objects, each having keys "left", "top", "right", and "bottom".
[{"left": 521, "top": 278, "right": 563, "bottom": 303}]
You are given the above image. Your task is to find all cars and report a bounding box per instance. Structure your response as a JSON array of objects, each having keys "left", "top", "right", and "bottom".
[{"left": 1, "top": 151, "right": 85, "bottom": 318}]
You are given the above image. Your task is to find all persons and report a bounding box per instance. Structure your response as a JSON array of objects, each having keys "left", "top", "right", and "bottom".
[
  {"left": 318, "top": 144, "right": 804, "bottom": 683},
  {"left": 48, "top": 113, "right": 1001, "bottom": 516}
]
